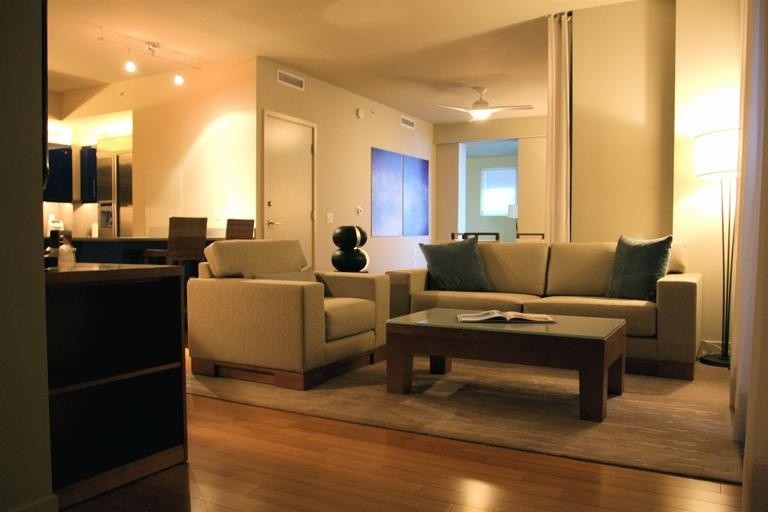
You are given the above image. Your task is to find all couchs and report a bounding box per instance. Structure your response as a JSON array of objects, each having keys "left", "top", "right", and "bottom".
[{"left": 385, "top": 239, "right": 701, "bottom": 380}]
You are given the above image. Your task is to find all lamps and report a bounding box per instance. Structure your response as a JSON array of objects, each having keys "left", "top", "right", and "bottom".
[
  {"left": 506, "top": 204, "right": 517, "bottom": 232},
  {"left": 93, "top": 24, "right": 201, "bottom": 86},
  {"left": 685, "top": 122, "right": 745, "bottom": 372},
  {"left": 469, "top": 110, "right": 491, "bottom": 119}
]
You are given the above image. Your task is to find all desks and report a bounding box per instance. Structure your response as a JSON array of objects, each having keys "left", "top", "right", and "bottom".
[{"left": 43, "top": 234, "right": 228, "bottom": 347}]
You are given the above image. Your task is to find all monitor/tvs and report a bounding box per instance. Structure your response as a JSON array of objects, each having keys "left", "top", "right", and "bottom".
[{"left": 42, "top": 147, "right": 73, "bottom": 204}]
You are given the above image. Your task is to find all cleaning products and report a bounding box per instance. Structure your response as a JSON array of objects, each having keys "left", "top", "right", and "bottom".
[{"left": 55, "top": 236, "right": 77, "bottom": 270}]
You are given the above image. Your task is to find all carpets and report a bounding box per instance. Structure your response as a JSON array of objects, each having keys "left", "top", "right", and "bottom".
[{"left": 181, "top": 359, "right": 748, "bottom": 487}]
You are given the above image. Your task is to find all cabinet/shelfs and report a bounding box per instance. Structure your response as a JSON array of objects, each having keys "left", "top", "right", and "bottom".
[{"left": 46, "top": 259, "right": 189, "bottom": 511}]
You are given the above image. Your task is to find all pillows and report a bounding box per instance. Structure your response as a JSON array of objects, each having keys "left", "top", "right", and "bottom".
[
  {"left": 417, "top": 236, "right": 498, "bottom": 292},
  {"left": 607, "top": 231, "right": 673, "bottom": 301}
]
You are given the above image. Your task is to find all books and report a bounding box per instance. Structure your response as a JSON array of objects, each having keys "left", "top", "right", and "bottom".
[{"left": 456, "top": 310, "right": 557, "bottom": 324}]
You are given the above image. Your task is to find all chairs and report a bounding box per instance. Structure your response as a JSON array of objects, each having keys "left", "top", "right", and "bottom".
[
  {"left": 141, "top": 218, "right": 207, "bottom": 265},
  {"left": 189, "top": 238, "right": 390, "bottom": 391},
  {"left": 223, "top": 218, "right": 254, "bottom": 238}
]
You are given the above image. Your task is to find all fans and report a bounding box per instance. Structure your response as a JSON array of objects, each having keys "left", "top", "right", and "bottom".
[{"left": 435, "top": 83, "right": 533, "bottom": 125}]
